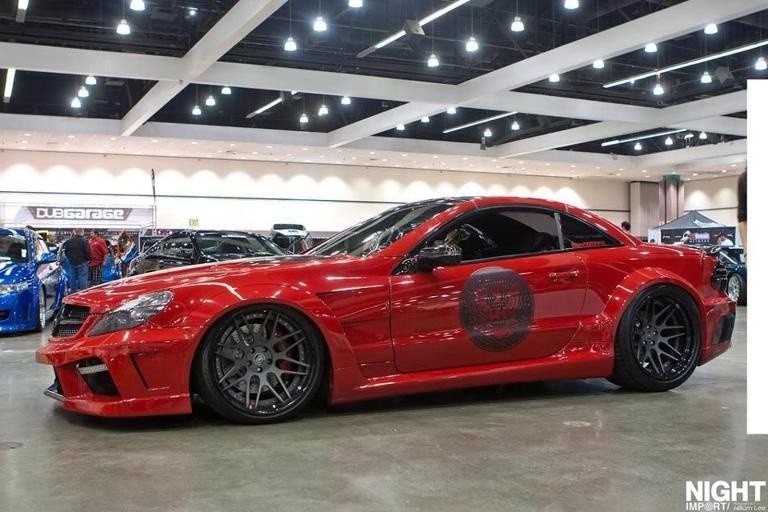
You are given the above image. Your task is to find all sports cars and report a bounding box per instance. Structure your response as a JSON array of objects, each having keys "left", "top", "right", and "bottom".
[{"left": 31, "top": 187, "right": 743, "bottom": 431}]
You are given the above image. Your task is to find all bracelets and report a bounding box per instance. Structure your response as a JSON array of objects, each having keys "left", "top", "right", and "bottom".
[{"left": 743, "top": 253, "right": 747, "bottom": 258}]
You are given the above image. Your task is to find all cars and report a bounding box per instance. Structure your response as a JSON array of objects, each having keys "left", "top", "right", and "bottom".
[
  {"left": 121, "top": 222, "right": 313, "bottom": 279},
  {"left": 46, "top": 232, "right": 138, "bottom": 286},
  {"left": 1, "top": 223, "right": 71, "bottom": 339},
  {"left": 687, "top": 243, "right": 746, "bottom": 307}
]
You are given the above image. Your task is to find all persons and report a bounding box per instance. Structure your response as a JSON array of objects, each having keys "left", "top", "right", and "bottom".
[
  {"left": 620, "top": 221, "right": 631, "bottom": 232},
  {"left": 650, "top": 239, "right": 655, "bottom": 243},
  {"left": 736, "top": 167, "right": 747, "bottom": 263},
  {"left": 718, "top": 233, "right": 733, "bottom": 246},
  {"left": 87, "top": 230, "right": 108, "bottom": 288},
  {"left": 65, "top": 227, "right": 92, "bottom": 293},
  {"left": 683, "top": 233, "right": 696, "bottom": 244}
]
